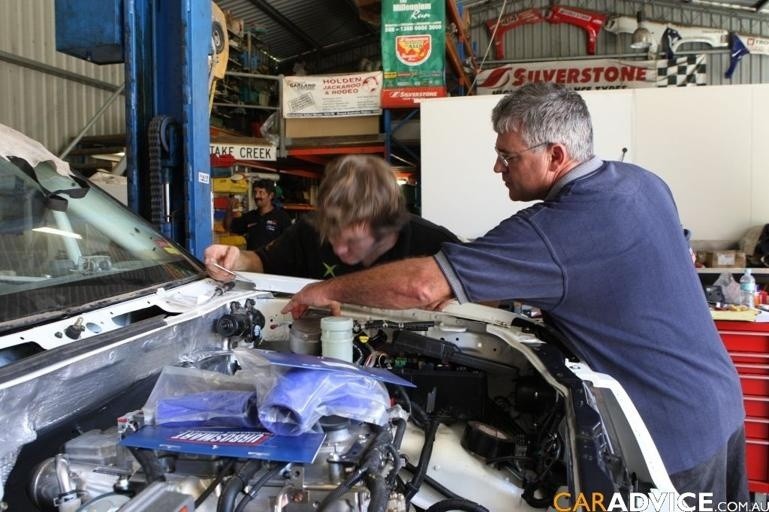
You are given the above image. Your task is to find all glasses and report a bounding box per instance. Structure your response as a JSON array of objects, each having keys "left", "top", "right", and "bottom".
[{"left": 499, "top": 140, "right": 552, "bottom": 165}]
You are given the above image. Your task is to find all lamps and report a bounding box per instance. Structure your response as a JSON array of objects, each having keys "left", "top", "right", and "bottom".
[{"left": 627, "top": 6, "right": 652, "bottom": 50}]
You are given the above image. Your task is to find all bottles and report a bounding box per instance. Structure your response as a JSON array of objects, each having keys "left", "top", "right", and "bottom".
[
  {"left": 321, "top": 315, "right": 354, "bottom": 367},
  {"left": 739, "top": 268, "right": 755, "bottom": 308}
]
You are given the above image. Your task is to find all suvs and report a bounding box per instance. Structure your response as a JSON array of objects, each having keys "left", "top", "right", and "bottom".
[{"left": 2, "top": 115, "right": 691, "bottom": 512}]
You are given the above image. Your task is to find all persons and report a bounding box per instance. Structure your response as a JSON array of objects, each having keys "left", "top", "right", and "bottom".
[
  {"left": 202, "top": 154, "right": 463, "bottom": 283},
  {"left": 223, "top": 179, "right": 291, "bottom": 252},
  {"left": 282, "top": 81, "right": 749, "bottom": 510}
]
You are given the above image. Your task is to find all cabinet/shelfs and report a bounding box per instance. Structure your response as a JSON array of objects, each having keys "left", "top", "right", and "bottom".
[{"left": 699, "top": 307, "right": 769, "bottom": 494}]
